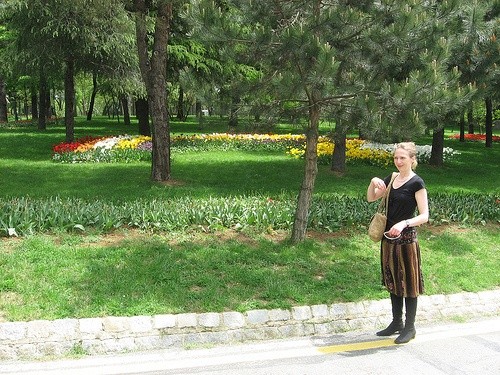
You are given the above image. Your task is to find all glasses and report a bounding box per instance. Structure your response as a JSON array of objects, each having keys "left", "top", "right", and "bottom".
[{"left": 383, "top": 231, "right": 402, "bottom": 240}]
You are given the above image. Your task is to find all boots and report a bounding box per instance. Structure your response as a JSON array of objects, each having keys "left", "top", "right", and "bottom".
[
  {"left": 376, "top": 294, "right": 404, "bottom": 337},
  {"left": 394, "top": 296, "right": 419, "bottom": 343}
]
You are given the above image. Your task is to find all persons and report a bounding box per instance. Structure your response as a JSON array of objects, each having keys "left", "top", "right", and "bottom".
[{"left": 366, "top": 142, "right": 429, "bottom": 344}]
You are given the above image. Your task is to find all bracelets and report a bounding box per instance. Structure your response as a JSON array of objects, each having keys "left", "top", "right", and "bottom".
[{"left": 404, "top": 219, "right": 409, "bottom": 229}]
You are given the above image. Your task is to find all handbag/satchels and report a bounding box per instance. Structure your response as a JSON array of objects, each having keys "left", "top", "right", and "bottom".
[{"left": 367, "top": 211, "right": 388, "bottom": 243}]
